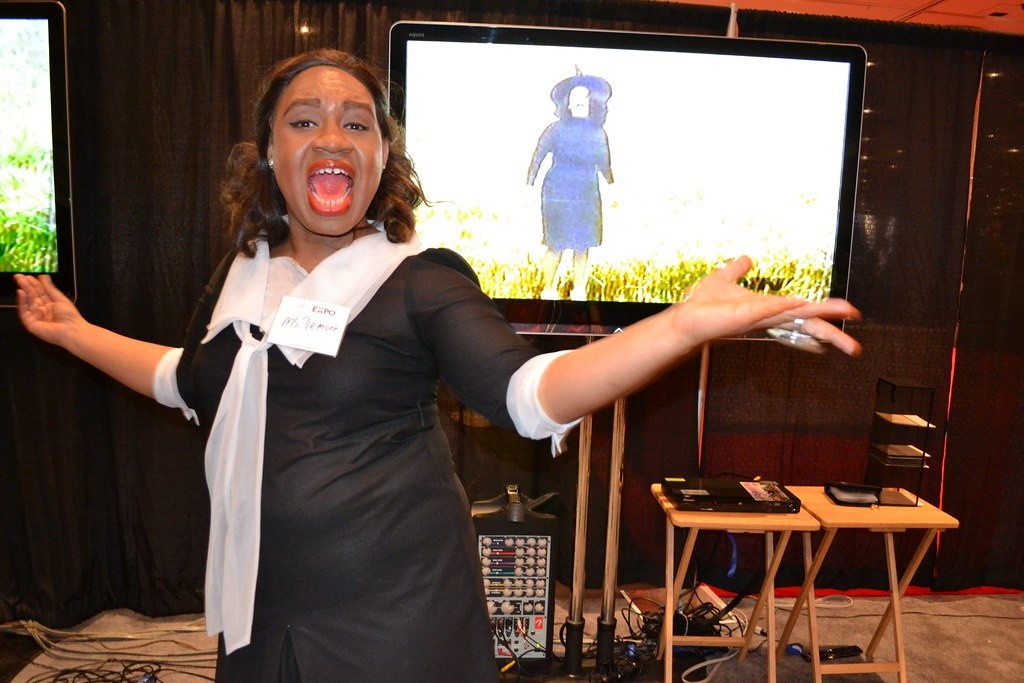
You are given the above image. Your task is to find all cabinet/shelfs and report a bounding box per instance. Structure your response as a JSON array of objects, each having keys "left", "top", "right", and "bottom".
[{"left": 862, "top": 376, "right": 937, "bottom": 507}]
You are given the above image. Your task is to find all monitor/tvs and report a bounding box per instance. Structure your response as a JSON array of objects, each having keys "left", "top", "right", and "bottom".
[
  {"left": 388, "top": 21, "right": 868, "bottom": 337},
  {"left": 0, "top": 0, "right": 78, "bottom": 308}
]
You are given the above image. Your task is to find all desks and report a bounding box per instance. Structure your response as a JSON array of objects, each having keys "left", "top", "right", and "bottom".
[
  {"left": 774, "top": 487, "right": 959, "bottom": 683},
  {"left": 650, "top": 483, "right": 821, "bottom": 683}
]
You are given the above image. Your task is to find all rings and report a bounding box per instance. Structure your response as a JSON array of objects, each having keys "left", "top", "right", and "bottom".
[{"left": 793, "top": 315, "right": 806, "bottom": 333}]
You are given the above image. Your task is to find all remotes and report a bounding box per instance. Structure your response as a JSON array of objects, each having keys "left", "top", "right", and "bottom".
[{"left": 800, "top": 645, "right": 863, "bottom": 663}]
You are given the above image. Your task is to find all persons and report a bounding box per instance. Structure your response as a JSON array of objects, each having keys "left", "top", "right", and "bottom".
[{"left": 15, "top": 50, "right": 864, "bottom": 683}]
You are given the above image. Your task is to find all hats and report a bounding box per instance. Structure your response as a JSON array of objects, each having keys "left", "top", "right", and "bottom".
[{"left": 550, "top": 65, "right": 613, "bottom": 104}]
